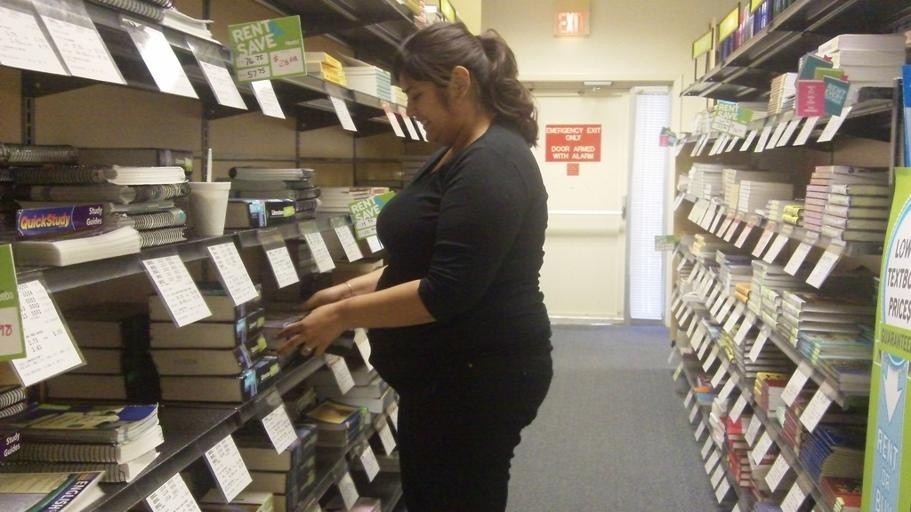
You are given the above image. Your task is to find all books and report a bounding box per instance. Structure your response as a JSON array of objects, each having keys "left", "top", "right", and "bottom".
[
  {"left": 262, "top": 309, "right": 306, "bottom": 360},
  {"left": 194, "top": 423, "right": 318, "bottom": 511},
  {"left": 691, "top": 234, "right": 737, "bottom": 264},
  {"left": 1, "top": 384, "right": 163, "bottom": 511},
  {"left": 736, "top": 340, "right": 788, "bottom": 378},
  {"left": 148, "top": 282, "right": 279, "bottom": 401},
  {"left": 215, "top": 166, "right": 320, "bottom": 230},
  {"left": 284, "top": 388, "right": 362, "bottom": 447},
  {"left": 38, "top": 302, "right": 126, "bottom": 399},
  {"left": 350, "top": 67, "right": 409, "bottom": 106},
  {"left": 753, "top": 370, "right": 816, "bottom": 417},
  {"left": 767, "top": 34, "right": 905, "bottom": 120},
  {"left": 715, "top": 249, "right": 751, "bottom": 295},
  {"left": 818, "top": 358, "right": 872, "bottom": 394},
  {"left": 90, "top": 1, "right": 214, "bottom": 42},
  {"left": 782, "top": 401, "right": 866, "bottom": 480},
  {"left": 337, "top": 257, "right": 381, "bottom": 279},
  {"left": 747, "top": 258, "right": 810, "bottom": 331},
  {"left": 306, "top": 51, "right": 346, "bottom": 87},
  {"left": 683, "top": 164, "right": 792, "bottom": 213},
  {"left": 2, "top": 142, "right": 193, "bottom": 268},
  {"left": 784, "top": 291, "right": 876, "bottom": 357},
  {"left": 783, "top": 164, "right": 891, "bottom": 244},
  {"left": 315, "top": 186, "right": 391, "bottom": 215},
  {"left": 315, "top": 367, "right": 397, "bottom": 412},
  {"left": 710, "top": 411, "right": 793, "bottom": 490}
]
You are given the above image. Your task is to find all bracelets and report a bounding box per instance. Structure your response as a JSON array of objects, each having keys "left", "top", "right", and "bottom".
[{"left": 347, "top": 281, "right": 354, "bottom": 299}]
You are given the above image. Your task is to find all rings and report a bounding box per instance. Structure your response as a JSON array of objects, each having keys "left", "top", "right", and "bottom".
[{"left": 305, "top": 346, "right": 312, "bottom": 352}]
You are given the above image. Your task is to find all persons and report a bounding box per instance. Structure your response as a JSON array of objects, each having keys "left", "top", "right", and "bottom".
[{"left": 275, "top": 21, "right": 556, "bottom": 511}]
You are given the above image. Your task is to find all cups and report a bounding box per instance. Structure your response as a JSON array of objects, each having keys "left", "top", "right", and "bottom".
[{"left": 187, "top": 181, "right": 232, "bottom": 236}]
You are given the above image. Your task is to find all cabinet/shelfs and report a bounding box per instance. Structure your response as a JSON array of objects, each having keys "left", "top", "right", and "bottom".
[
  {"left": 0, "top": 0, "right": 423, "bottom": 512},
  {"left": 665, "top": 0, "right": 911, "bottom": 512}
]
